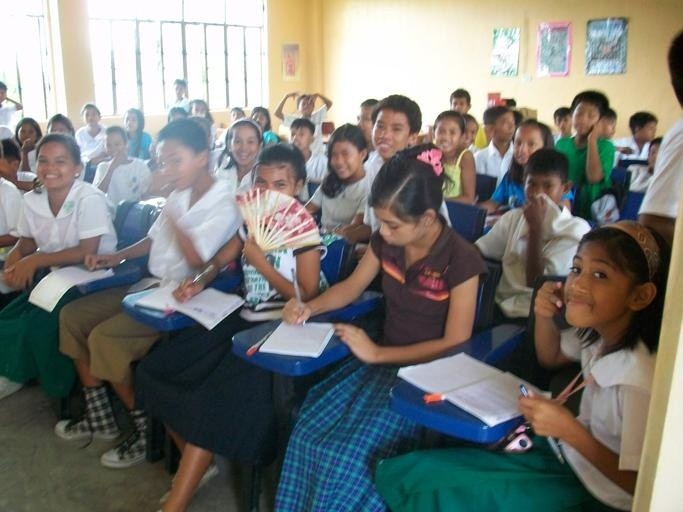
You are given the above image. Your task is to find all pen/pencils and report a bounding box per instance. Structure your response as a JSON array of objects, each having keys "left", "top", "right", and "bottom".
[
  {"left": 246, "top": 329, "right": 273, "bottom": 356},
  {"left": 291, "top": 268, "right": 305, "bottom": 327},
  {"left": 177, "top": 264, "right": 214, "bottom": 298},
  {"left": 519, "top": 384, "right": 565, "bottom": 465}
]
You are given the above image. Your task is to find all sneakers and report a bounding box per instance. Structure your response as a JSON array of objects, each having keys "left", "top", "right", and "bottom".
[
  {"left": 0, "top": 376, "right": 25, "bottom": 400},
  {"left": 158, "top": 461, "right": 221, "bottom": 506}
]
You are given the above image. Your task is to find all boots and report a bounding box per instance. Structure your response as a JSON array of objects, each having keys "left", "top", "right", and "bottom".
[
  {"left": 50, "top": 381, "right": 121, "bottom": 444},
  {"left": 97, "top": 409, "right": 157, "bottom": 470}
]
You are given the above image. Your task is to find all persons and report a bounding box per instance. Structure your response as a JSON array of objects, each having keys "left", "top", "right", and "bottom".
[
  {"left": 617, "top": 106, "right": 659, "bottom": 164},
  {"left": 1, "top": 75, "right": 616, "bottom": 512},
  {"left": 370, "top": 218, "right": 671, "bottom": 512},
  {"left": 633, "top": 26, "right": 683, "bottom": 214},
  {"left": 618, "top": 134, "right": 662, "bottom": 219}
]
